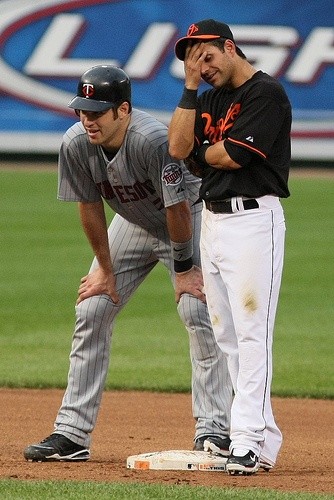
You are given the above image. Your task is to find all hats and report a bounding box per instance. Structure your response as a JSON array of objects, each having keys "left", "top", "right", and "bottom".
[{"left": 174, "top": 19, "right": 235, "bottom": 61}]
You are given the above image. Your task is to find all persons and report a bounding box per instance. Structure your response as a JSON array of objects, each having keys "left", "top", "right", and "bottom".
[
  {"left": 168, "top": 20, "right": 293, "bottom": 477},
  {"left": 24, "top": 65, "right": 236, "bottom": 464}
]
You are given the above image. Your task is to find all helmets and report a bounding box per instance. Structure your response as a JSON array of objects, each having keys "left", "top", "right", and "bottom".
[{"left": 68, "top": 65, "right": 131, "bottom": 117}]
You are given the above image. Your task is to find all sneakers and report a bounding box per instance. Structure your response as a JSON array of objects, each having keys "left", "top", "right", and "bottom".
[
  {"left": 193, "top": 436, "right": 208, "bottom": 451},
  {"left": 226, "top": 448, "right": 260, "bottom": 476},
  {"left": 205, "top": 436, "right": 273, "bottom": 471},
  {"left": 24, "top": 433, "right": 90, "bottom": 462}
]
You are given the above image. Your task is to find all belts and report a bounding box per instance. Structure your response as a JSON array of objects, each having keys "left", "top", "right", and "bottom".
[{"left": 205, "top": 198, "right": 259, "bottom": 214}]
